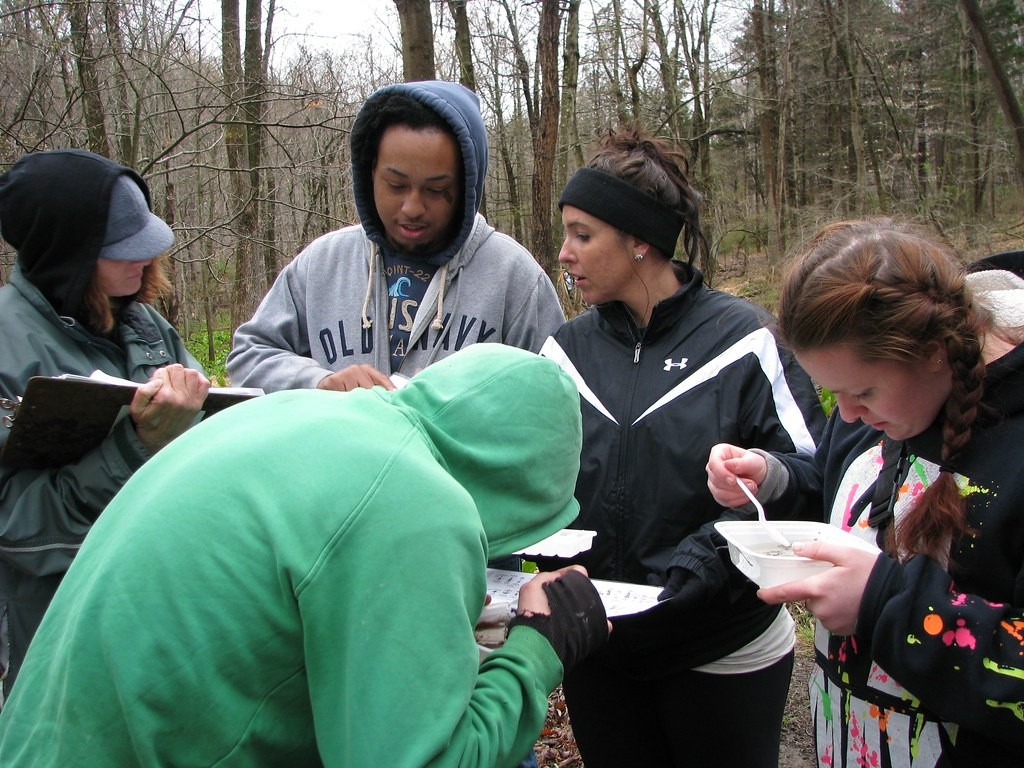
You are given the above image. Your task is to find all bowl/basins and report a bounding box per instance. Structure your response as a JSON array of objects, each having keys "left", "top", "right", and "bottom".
[{"left": 714, "top": 522, "right": 881, "bottom": 589}]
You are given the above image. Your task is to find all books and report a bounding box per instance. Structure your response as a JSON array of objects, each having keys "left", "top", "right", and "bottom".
[{"left": 0, "top": 370, "right": 266, "bottom": 487}]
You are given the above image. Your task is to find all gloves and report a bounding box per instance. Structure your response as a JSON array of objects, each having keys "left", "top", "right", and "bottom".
[{"left": 646, "top": 566, "right": 712, "bottom": 614}]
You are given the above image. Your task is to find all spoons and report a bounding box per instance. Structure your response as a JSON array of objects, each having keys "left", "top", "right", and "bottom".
[{"left": 736, "top": 477, "right": 790, "bottom": 547}]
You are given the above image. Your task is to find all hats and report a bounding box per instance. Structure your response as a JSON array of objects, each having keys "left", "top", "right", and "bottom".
[{"left": 97, "top": 173, "right": 175, "bottom": 262}]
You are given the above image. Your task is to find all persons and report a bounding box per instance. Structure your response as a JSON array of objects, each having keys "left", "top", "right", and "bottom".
[
  {"left": 706, "top": 218, "right": 1024, "bottom": 768},
  {"left": 536, "top": 131, "right": 829, "bottom": 768},
  {"left": 0, "top": 343, "right": 614, "bottom": 768},
  {"left": 0, "top": 147, "right": 211, "bottom": 712},
  {"left": 225, "top": 82, "right": 567, "bottom": 768}
]
